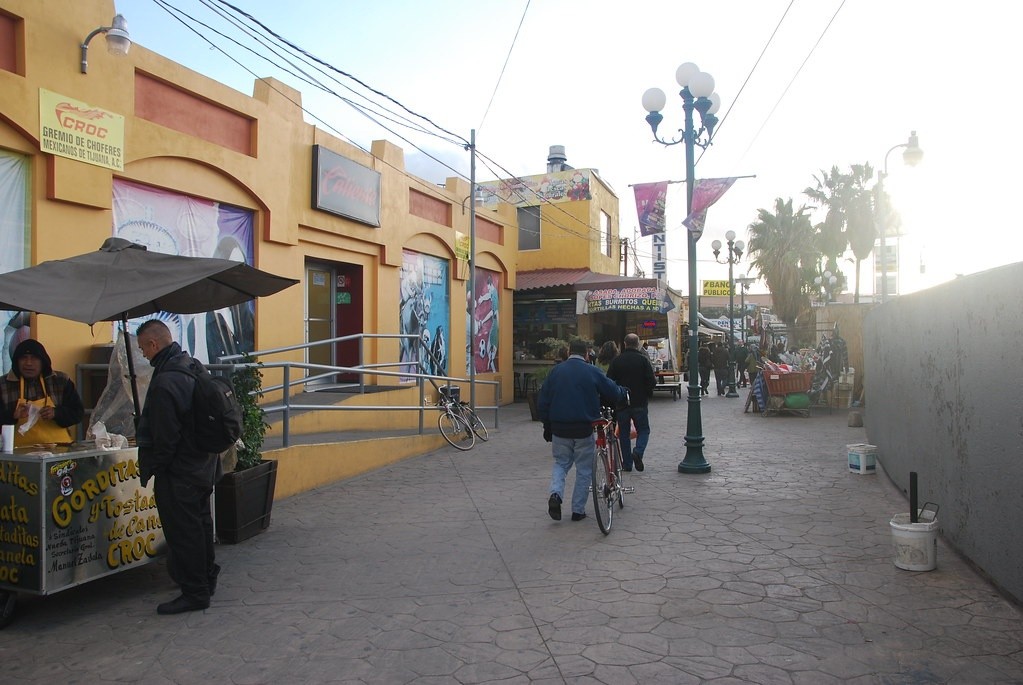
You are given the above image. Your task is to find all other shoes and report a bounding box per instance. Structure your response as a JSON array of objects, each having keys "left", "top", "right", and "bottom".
[
  {"left": 716, "top": 387, "right": 726, "bottom": 396},
  {"left": 571, "top": 511, "right": 587, "bottom": 521},
  {"left": 157, "top": 595, "right": 210, "bottom": 615},
  {"left": 548, "top": 493, "right": 562, "bottom": 520},
  {"left": 622, "top": 462, "right": 632, "bottom": 472},
  {"left": 701, "top": 389, "right": 709, "bottom": 395},
  {"left": 631, "top": 453, "right": 644, "bottom": 472}
]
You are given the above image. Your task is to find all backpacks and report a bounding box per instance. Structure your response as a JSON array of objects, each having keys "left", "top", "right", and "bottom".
[{"left": 154, "top": 358, "right": 244, "bottom": 455}]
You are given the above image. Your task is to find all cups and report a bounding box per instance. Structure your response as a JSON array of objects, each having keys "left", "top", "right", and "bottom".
[{"left": 2, "top": 424, "right": 14, "bottom": 454}]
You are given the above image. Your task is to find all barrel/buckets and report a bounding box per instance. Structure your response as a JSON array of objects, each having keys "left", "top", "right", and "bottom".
[
  {"left": 890, "top": 508, "right": 940, "bottom": 571},
  {"left": 846, "top": 443, "right": 877, "bottom": 475}
]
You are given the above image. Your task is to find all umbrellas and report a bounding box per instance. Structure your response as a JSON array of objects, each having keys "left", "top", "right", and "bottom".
[{"left": 0, "top": 237, "right": 301, "bottom": 415}]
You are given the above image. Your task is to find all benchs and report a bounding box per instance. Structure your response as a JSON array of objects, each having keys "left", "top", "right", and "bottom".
[{"left": 654, "top": 385, "right": 682, "bottom": 401}]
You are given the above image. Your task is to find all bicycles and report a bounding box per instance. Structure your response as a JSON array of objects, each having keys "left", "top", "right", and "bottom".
[
  {"left": 589, "top": 390, "right": 636, "bottom": 536},
  {"left": 438, "top": 382, "right": 491, "bottom": 452}
]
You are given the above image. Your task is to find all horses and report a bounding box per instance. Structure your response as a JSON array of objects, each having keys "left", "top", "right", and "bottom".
[{"left": 400, "top": 289, "right": 434, "bottom": 360}]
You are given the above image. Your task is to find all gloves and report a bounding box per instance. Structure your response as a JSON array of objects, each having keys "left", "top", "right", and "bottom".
[{"left": 541, "top": 423, "right": 554, "bottom": 443}]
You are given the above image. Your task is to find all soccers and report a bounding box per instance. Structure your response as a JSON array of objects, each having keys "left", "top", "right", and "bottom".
[{"left": 479, "top": 339, "right": 486, "bottom": 358}]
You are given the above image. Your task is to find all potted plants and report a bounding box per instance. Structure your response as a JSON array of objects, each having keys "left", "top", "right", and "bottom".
[
  {"left": 526, "top": 365, "right": 553, "bottom": 421},
  {"left": 225, "top": 330, "right": 278, "bottom": 546}
]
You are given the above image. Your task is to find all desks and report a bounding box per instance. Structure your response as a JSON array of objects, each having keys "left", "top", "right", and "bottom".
[{"left": 655, "top": 372, "right": 681, "bottom": 384}]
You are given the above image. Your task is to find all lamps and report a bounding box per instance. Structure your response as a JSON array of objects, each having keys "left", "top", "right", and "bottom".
[
  {"left": 462, "top": 186, "right": 484, "bottom": 215},
  {"left": 81, "top": 16, "right": 130, "bottom": 73}
]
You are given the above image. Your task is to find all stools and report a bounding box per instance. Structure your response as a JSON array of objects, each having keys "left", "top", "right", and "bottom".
[
  {"left": 524, "top": 373, "right": 538, "bottom": 398},
  {"left": 514, "top": 372, "right": 522, "bottom": 399}
]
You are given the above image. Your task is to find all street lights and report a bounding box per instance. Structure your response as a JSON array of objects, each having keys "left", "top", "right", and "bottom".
[
  {"left": 711, "top": 229, "right": 746, "bottom": 399},
  {"left": 877, "top": 129, "right": 925, "bottom": 300},
  {"left": 737, "top": 273, "right": 746, "bottom": 342},
  {"left": 642, "top": 60, "right": 721, "bottom": 474},
  {"left": 814, "top": 269, "right": 839, "bottom": 306}
]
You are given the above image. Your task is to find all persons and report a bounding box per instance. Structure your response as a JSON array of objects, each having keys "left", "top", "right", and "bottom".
[
  {"left": 734, "top": 339, "right": 793, "bottom": 389},
  {"left": 562, "top": 346, "right": 569, "bottom": 361},
  {"left": 136, "top": 318, "right": 221, "bottom": 615},
  {"left": 187, "top": 234, "right": 255, "bottom": 372},
  {"left": 712, "top": 342, "right": 731, "bottom": 395},
  {"left": 0, "top": 339, "right": 86, "bottom": 447},
  {"left": 400, "top": 272, "right": 446, "bottom": 382},
  {"left": 538, "top": 341, "right": 631, "bottom": 522},
  {"left": 659, "top": 352, "right": 666, "bottom": 384},
  {"left": 643, "top": 342, "right": 659, "bottom": 372},
  {"left": 698, "top": 343, "right": 711, "bottom": 395},
  {"left": 587, "top": 344, "right": 596, "bottom": 366},
  {"left": 465, "top": 291, "right": 484, "bottom": 374},
  {"left": 607, "top": 334, "right": 657, "bottom": 472},
  {"left": 596, "top": 341, "right": 620, "bottom": 373},
  {"left": 478, "top": 275, "right": 499, "bottom": 373}
]
